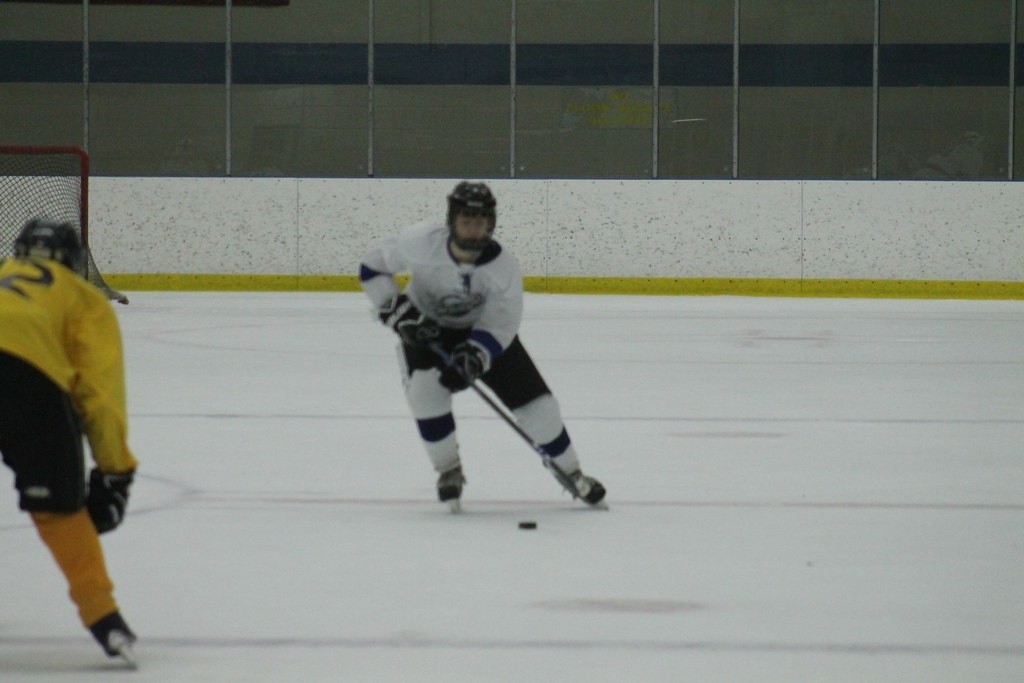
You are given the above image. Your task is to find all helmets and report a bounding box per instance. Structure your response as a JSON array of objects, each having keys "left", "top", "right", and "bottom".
[
  {"left": 448, "top": 182, "right": 497, "bottom": 232},
  {"left": 14, "top": 220, "right": 81, "bottom": 272}
]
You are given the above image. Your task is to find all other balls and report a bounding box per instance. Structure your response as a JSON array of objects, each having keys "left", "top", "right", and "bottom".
[{"left": 518, "top": 520, "right": 539, "bottom": 530}]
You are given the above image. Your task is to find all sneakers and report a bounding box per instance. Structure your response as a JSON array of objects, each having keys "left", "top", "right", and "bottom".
[
  {"left": 438, "top": 465, "right": 463, "bottom": 513},
  {"left": 91, "top": 611, "right": 138, "bottom": 669},
  {"left": 564, "top": 470, "right": 608, "bottom": 512}
]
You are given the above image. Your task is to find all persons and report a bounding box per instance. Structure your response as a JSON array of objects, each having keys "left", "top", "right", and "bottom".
[
  {"left": 0, "top": 218, "right": 142, "bottom": 669},
  {"left": 358, "top": 180, "right": 610, "bottom": 514}
]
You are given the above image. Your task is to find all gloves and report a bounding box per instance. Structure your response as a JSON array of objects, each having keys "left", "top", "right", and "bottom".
[
  {"left": 398, "top": 313, "right": 449, "bottom": 363},
  {"left": 87, "top": 468, "right": 133, "bottom": 534},
  {"left": 440, "top": 342, "right": 486, "bottom": 393}
]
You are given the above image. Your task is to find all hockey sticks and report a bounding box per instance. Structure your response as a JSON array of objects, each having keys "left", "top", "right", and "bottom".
[{"left": 422, "top": 338, "right": 581, "bottom": 499}]
